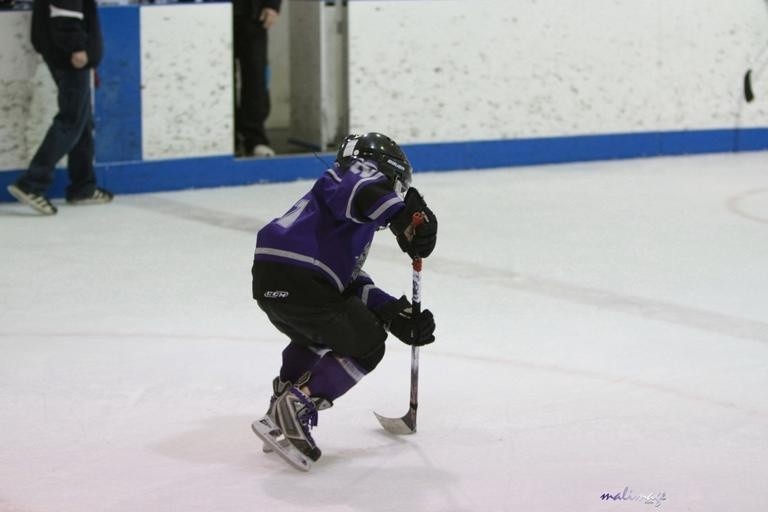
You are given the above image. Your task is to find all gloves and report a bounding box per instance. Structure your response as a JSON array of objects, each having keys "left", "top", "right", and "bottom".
[
  {"left": 380, "top": 294, "right": 435, "bottom": 346},
  {"left": 389, "top": 187, "right": 437, "bottom": 258}
]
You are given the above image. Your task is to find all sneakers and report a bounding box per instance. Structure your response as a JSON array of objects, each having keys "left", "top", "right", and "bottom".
[
  {"left": 7, "top": 179, "right": 57, "bottom": 215},
  {"left": 65, "top": 187, "right": 113, "bottom": 204},
  {"left": 253, "top": 145, "right": 274, "bottom": 156}
]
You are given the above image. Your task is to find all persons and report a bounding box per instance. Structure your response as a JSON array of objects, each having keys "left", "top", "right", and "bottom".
[
  {"left": 250, "top": 131, "right": 439, "bottom": 462},
  {"left": 205, "top": 0, "right": 283, "bottom": 158},
  {"left": 7, "top": 0, "right": 115, "bottom": 216}
]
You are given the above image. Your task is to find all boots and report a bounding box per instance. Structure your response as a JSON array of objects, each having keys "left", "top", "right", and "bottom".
[{"left": 267, "top": 370, "right": 333, "bottom": 461}]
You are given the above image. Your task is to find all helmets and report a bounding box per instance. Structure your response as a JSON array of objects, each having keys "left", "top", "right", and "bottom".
[{"left": 336, "top": 132, "right": 412, "bottom": 193}]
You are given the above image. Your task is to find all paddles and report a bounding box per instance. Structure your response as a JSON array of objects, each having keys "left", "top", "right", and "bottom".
[{"left": 373, "top": 212, "right": 423, "bottom": 436}]
